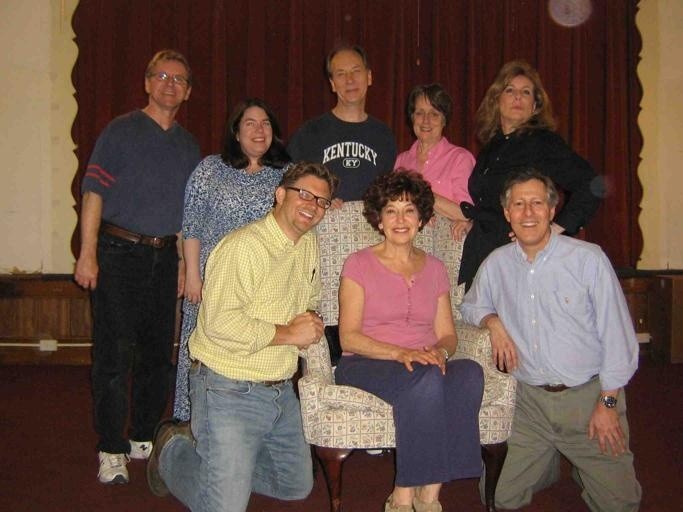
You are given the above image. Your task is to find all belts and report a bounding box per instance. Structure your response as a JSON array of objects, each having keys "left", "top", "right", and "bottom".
[
  {"left": 105, "top": 225, "right": 173, "bottom": 248},
  {"left": 539, "top": 384, "right": 567, "bottom": 392}
]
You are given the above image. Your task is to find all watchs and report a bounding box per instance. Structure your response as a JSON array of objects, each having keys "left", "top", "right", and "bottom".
[
  {"left": 432, "top": 344, "right": 449, "bottom": 361},
  {"left": 598, "top": 393, "right": 616, "bottom": 409}
]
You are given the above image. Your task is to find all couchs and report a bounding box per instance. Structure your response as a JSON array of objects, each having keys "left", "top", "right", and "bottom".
[{"left": 290, "top": 194, "right": 517, "bottom": 511}]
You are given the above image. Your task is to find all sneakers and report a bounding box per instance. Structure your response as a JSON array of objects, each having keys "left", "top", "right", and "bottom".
[
  {"left": 97, "top": 420, "right": 183, "bottom": 499},
  {"left": 385, "top": 487, "right": 443, "bottom": 512}
]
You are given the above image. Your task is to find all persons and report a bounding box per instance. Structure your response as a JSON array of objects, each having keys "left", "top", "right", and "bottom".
[
  {"left": 455, "top": 169, "right": 643, "bottom": 511},
  {"left": 172, "top": 98, "right": 298, "bottom": 423},
  {"left": 144, "top": 161, "right": 338, "bottom": 510},
  {"left": 73, "top": 50, "right": 209, "bottom": 484},
  {"left": 458, "top": 61, "right": 601, "bottom": 294},
  {"left": 288, "top": 46, "right": 398, "bottom": 199},
  {"left": 392, "top": 84, "right": 474, "bottom": 224},
  {"left": 333, "top": 168, "right": 482, "bottom": 510}
]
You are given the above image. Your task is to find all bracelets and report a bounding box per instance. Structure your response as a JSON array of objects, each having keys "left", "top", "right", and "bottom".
[{"left": 308, "top": 309, "right": 323, "bottom": 319}]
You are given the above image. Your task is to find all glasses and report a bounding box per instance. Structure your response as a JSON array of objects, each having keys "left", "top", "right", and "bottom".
[
  {"left": 148, "top": 72, "right": 187, "bottom": 86},
  {"left": 286, "top": 186, "right": 331, "bottom": 209}
]
